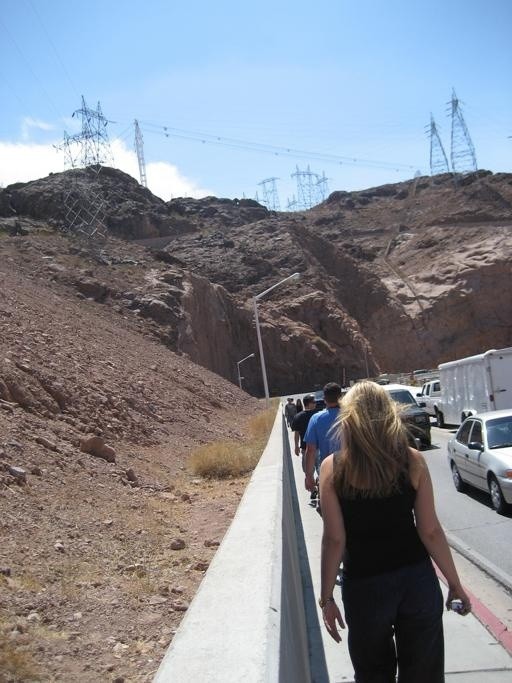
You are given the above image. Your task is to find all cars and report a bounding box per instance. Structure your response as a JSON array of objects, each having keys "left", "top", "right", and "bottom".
[
  {"left": 382, "top": 388, "right": 430, "bottom": 445},
  {"left": 376, "top": 374, "right": 389, "bottom": 384},
  {"left": 313, "top": 391, "right": 326, "bottom": 410},
  {"left": 447, "top": 409, "right": 512, "bottom": 510}
]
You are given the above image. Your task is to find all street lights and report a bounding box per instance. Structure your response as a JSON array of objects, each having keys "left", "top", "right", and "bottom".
[
  {"left": 252, "top": 273, "right": 302, "bottom": 404},
  {"left": 238, "top": 354, "right": 256, "bottom": 388}
]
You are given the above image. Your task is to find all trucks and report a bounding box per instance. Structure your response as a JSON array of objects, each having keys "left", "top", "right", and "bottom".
[{"left": 434, "top": 347, "right": 512, "bottom": 426}]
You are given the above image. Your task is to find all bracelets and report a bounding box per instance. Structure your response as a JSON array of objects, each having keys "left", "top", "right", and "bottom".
[{"left": 319, "top": 597, "right": 334, "bottom": 609}]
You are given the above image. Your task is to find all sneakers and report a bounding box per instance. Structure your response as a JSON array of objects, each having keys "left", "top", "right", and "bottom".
[{"left": 310, "top": 486, "right": 318, "bottom": 499}]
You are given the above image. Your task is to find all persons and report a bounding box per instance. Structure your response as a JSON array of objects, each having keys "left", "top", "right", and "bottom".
[
  {"left": 312, "top": 377, "right": 471, "bottom": 683},
  {"left": 285, "top": 397, "right": 296, "bottom": 426},
  {"left": 302, "top": 381, "right": 346, "bottom": 587},
  {"left": 290, "top": 394, "right": 319, "bottom": 500},
  {"left": 296, "top": 398, "right": 303, "bottom": 413}
]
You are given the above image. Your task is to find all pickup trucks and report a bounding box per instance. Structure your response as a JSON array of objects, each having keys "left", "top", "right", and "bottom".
[{"left": 416, "top": 380, "right": 441, "bottom": 416}]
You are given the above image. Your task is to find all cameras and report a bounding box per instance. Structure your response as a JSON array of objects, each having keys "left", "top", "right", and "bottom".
[{"left": 451, "top": 601, "right": 465, "bottom": 614}]
